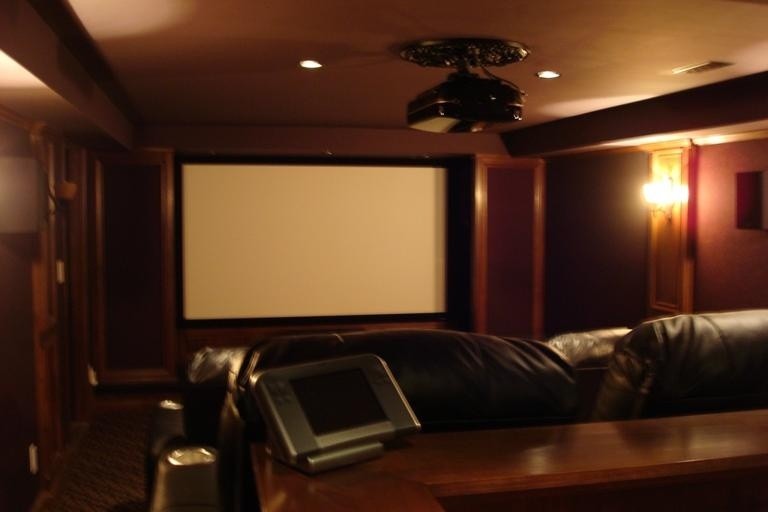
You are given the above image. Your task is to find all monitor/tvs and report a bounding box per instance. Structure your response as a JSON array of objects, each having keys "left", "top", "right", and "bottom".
[{"left": 251, "top": 355, "right": 421, "bottom": 463}]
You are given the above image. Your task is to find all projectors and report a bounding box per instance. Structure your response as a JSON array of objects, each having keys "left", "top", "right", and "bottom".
[{"left": 407, "top": 73, "right": 525, "bottom": 134}]
